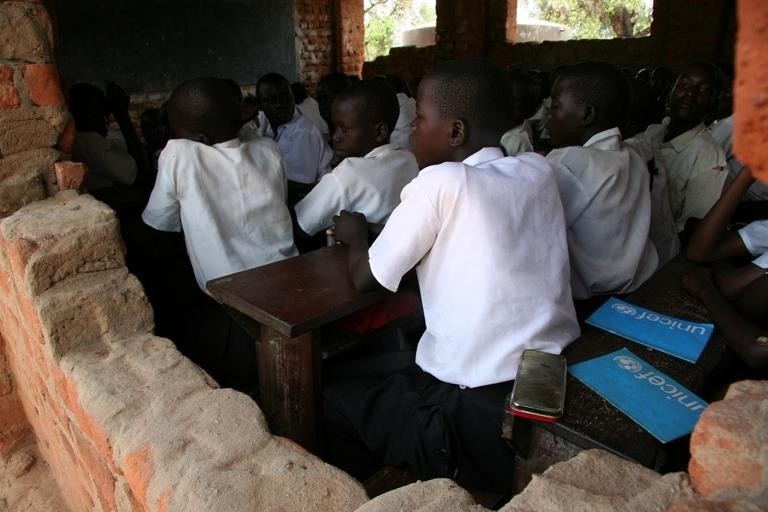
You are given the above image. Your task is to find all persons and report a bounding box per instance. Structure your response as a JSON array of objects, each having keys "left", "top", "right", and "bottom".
[
  {"left": 683, "top": 164, "right": 768, "bottom": 368},
  {"left": 66, "top": 72, "right": 334, "bottom": 388},
  {"left": 317, "top": 58, "right": 727, "bottom": 473}
]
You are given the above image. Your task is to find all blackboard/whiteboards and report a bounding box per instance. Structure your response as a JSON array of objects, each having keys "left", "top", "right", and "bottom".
[{"left": 53, "top": 0, "right": 297, "bottom": 97}]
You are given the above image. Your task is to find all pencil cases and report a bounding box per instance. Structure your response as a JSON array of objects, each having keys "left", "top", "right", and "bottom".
[{"left": 510, "top": 350, "right": 568, "bottom": 421}]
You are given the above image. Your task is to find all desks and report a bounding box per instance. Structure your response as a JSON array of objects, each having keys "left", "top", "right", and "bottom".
[
  {"left": 511, "top": 278, "right": 768, "bottom": 478},
  {"left": 202, "top": 227, "right": 425, "bottom": 449}
]
